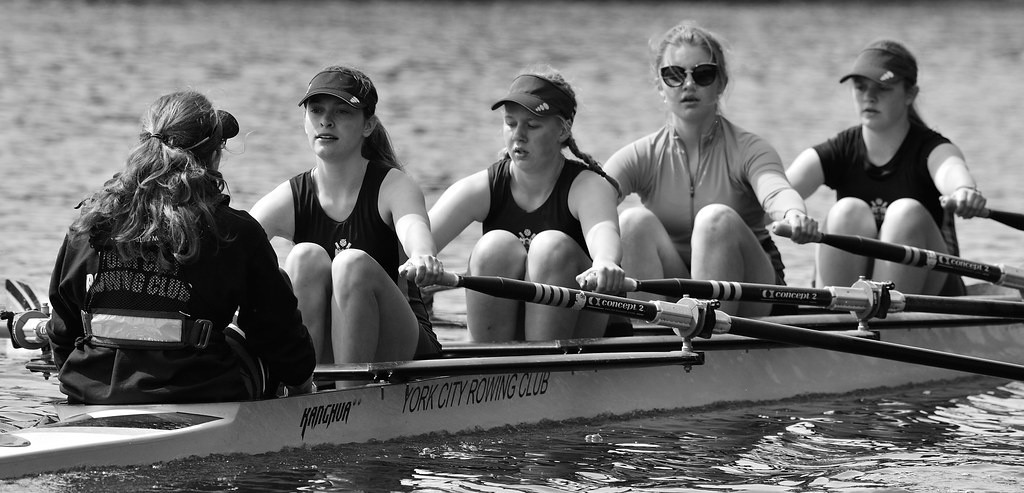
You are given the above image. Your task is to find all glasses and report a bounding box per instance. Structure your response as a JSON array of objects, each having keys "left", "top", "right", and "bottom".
[
  {"left": 220, "top": 138, "right": 227, "bottom": 149},
  {"left": 661, "top": 63, "right": 718, "bottom": 87}
]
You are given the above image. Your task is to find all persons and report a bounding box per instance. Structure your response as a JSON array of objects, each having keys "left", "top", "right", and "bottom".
[
  {"left": 249, "top": 66, "right": 444, "bottom": 389},
  {"left": 44, "top": 90, "right": 317, "bottom": 406},
  {"left": 785, "top": 39, "right": 987, "bottom": 297},
  {"left": 603, "top": 23, "right": 818, "bottom": 325},
  {"left": 428, "top": 66, "right": 624, "bottom": 344}
]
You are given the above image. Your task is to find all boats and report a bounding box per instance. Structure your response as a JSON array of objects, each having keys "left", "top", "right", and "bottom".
[{"left": 0, "top": 267, "right": 1024, "bottom": 484}]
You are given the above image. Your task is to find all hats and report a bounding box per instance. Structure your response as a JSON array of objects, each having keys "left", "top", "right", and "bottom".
[
  {"left": 490, "top": 74, "right": 574, "bottom": 118},
  {"left": 183, "top": 109, "right": 240, "bottom": 151},
  {"left": 839, "top": 48, "right": 913, "bottom": 86},
  {"left": 298, "top": 71, "right": 378, "bottom": 108}
]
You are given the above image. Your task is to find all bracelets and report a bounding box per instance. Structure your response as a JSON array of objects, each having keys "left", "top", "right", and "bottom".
[{"left": 954, "top": 186, "right": 981, "bottom": 193}]
